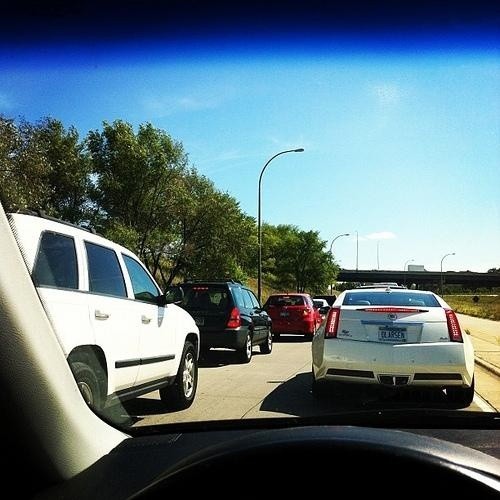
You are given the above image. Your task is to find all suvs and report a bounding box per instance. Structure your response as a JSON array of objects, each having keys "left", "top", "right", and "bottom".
[
  {"left": 166, "top": 277, "right": 273, "bottom": 363},
  {"left": 7, "top": 207, "right": 205, "bottom": 421}
]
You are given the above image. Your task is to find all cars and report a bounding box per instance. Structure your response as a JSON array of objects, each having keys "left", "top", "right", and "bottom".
[{"left": 261, "top": 282, "right": 477, "bottom": 410}]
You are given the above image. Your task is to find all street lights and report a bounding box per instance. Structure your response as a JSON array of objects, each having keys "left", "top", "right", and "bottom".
[
  {"left": 330, "top": 234, "right": 350, "bottom": 294},
  {"left": 441, "top": 252, "right": 456, "bottom": 297},
  {"left": 257, "top": 147, "right": 304, "bottom": 304},
  {"left": 353, "top": 229, "right": 358, "bottom": 271},
  {"left": 403, "top": 259, "right": 415, "bottom": 287}
]
